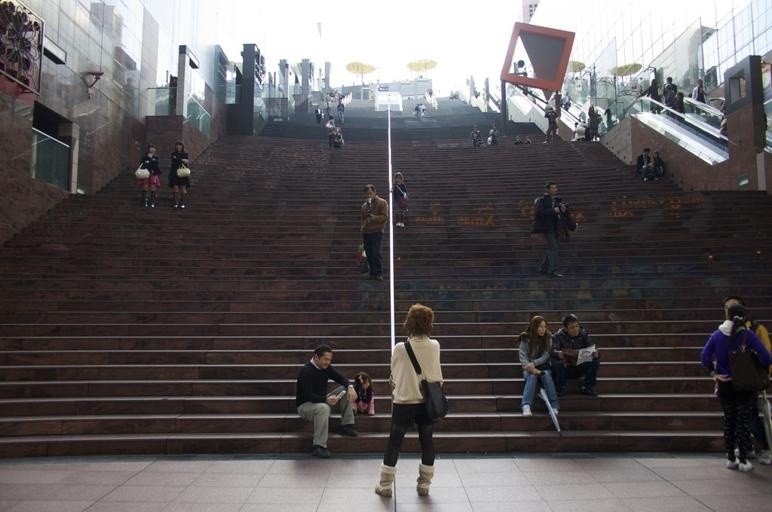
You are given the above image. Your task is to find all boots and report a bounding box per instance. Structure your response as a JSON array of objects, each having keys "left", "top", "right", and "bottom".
[
  {"left": 173, "top": 191, "right": 188, "bottom": 208},
  {"left": 144, "top": 190, "right": 157, "bottom": 208},
  {"left": 417, "top": 464, "right": 434, "bottom": 496},
  {"left": 375, "top": 464, "right": 397, "bottom": 496}
]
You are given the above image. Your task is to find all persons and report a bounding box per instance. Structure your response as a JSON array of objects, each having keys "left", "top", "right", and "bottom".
[
  {"left": 551, "top": 314, "right": 600, "bottom": 397},
  {"left": 637, "top": 148, "right": 663, "bottom": 180},
  {"left": 360, "top": 184, "right": 388, "bottom": 281},
  {"left": 353, "top": 372, "right": 376, "bottom": 416},
  {"left": 453, "top": 89, "right": 487, "bottom": 112},
  {"left": 416, "top": 89, "right": 438, "bottom": 117},
  {"left": 296, "top": 344, "right": 358, "bottom": 457},
  {"left": 518, "top": 316, "right": 561, "bottom": 416},
  {"left": 514, "top": 135, "right": 532, "bottom": 144},
  {"left": 524, "top": 88, "right": 536, "bottom": 104},
  {"left": 137, "top": 143, "right": 161, "bottom": 208},
  {"left": 532, "top": 181, "right": 576, "bottom": 277},
  {"left": 700, "top": 306, "right": 771, "bottom": 472},
  {"left": 471, "top": 125, "right": 498, "bottom": 146},
  {"left": 375, "top": 304, "right": 443, "bottom": 497},
  {"left": 167, "top": 141, "right": 191, "bottom": 210},
  {"left": 645, "top": 76, "right": 728, "bottom": 137},
  {"left": 544, "top": 90, "right": 612, "bottom": 144},
  {"left": 713, "top": 296, "right": 772, "bottom": 465},
  {"left": 392, "top": 171, "right": 408, "bottom": 227},
  {"left": 315, "top": 90, "right": 345, "bottom": 148}
]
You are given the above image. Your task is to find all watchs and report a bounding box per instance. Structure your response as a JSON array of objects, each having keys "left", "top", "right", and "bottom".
[{"left": 347, "top": 385, "right": 353, "bottom": 390}]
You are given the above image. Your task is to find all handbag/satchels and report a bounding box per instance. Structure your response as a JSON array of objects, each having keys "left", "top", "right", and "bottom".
[
  {"left": 729, "top": 349, "right": 767, "bottom": 393},
  {"left": 419, "top": 377, "right": 449, "bottom": 420},
  {"left": 177, "top": 164, "right": 190, "bottom": 178},
  {"left": 533, "top": 353, "right": 550, "bottom": 370},
  {"left": 135, "top": 164, "right": 150, "bottom": 179}
]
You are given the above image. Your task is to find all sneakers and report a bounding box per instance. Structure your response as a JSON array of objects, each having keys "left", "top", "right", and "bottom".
[
  {"left": 313, "top": 446, "right": 331, "bottom": 458},
  {"left": 727, "top": 447, "right": 771, "bottom": 472},
  {"left": 523, "top": 404, "right": 532, "bottom": 417},
  {"left": 579, "top": 386, "right": 597, "bottom": 396},
  {"left": 338, "top": 425, "right": 357, "bottom": 435}
]
